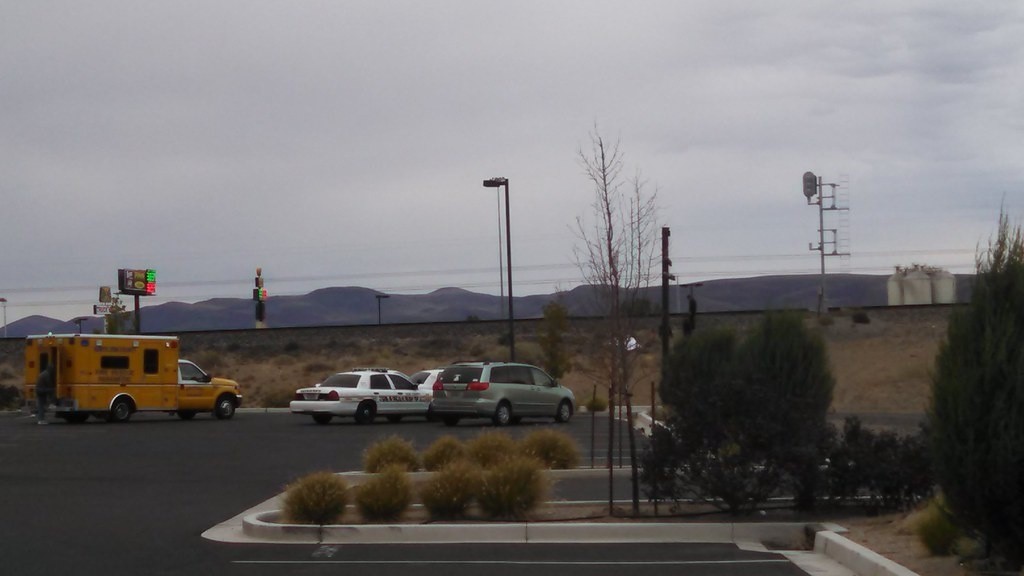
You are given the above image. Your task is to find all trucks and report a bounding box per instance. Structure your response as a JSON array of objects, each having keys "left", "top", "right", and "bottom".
[{"left": 22, "top": 331, "right": 243, "bottom": 422}]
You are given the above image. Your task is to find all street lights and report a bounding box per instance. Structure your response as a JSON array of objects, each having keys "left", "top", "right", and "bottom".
[{"left": 481, "top": 177, "right": 516, "bottom": 361}]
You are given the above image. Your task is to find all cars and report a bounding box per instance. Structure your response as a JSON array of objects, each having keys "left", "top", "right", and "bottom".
[
  {"left": 289, "top": 365, "right": 429, "bottom": 423},
  {"left": 408, "top": 369, "right": 481, "bottom": 399},
  {"left": 429, "top": 360, "right": 577, "bottom": 424}
]
